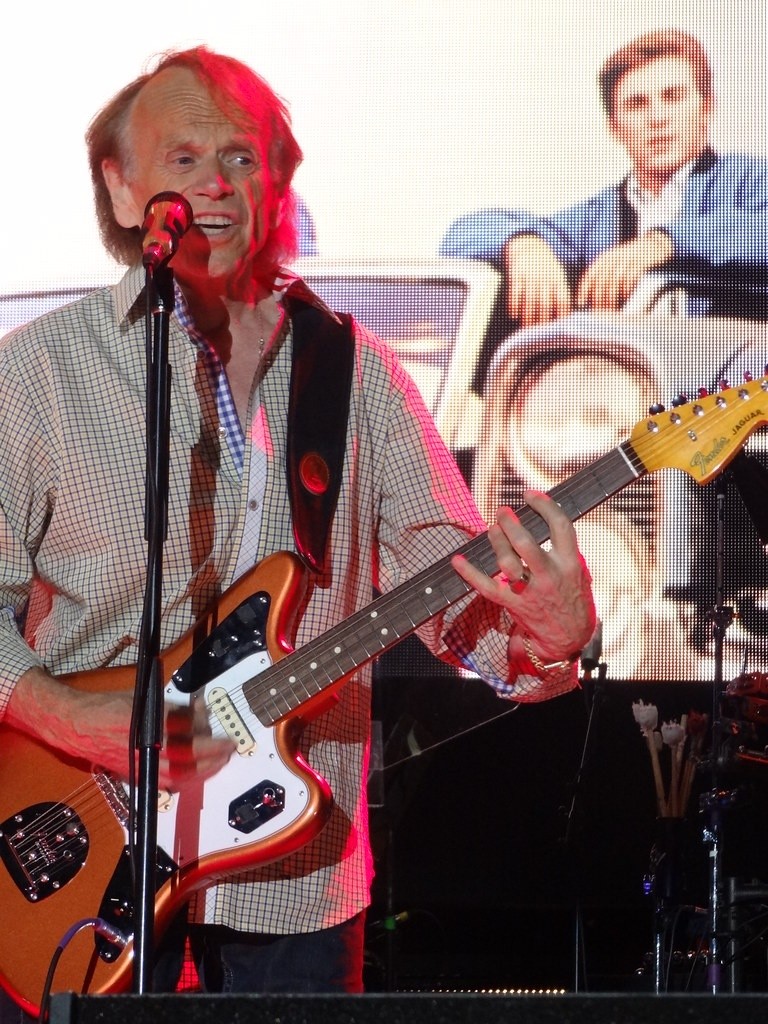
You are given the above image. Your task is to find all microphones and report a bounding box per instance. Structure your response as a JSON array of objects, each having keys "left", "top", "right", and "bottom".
[
  {"left": 581, "top": 619, "right": 602, "bottom": 671},
  {"left": 141, "top": 192, "right": 193, "bottom": 268},
  {"left": 367, "top": 911, "right": 410, "bottom": 942}
]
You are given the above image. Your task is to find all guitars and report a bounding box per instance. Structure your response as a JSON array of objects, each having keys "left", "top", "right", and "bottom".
[{"left": 0, "top": 361, "right": 768, "bottom": 1019}]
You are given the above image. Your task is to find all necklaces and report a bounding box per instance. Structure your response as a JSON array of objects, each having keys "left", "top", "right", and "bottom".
[{"left": 257, "top": 301, "right": 264, "bottom": 358}]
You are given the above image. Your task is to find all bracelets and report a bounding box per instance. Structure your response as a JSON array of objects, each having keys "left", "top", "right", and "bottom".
[{"left": 523, "top": 630, "right": 583, "bottom": 674}]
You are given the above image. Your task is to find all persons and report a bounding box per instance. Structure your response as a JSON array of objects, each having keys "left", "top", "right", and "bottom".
[
  {"left": 0, "top": 46, "right": 600, "bottom": 997},
  {"left": 442, "top": 30, "right": 768, "bottom": 334}
]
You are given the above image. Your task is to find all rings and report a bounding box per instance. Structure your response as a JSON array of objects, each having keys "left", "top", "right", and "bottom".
[{"left": 509, "top": 566, "right": 531, "bottom": 589}]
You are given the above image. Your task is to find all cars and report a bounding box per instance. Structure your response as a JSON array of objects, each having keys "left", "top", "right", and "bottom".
[{"left": 0, "top": 256, "right": 767, "bottom": 681}]
